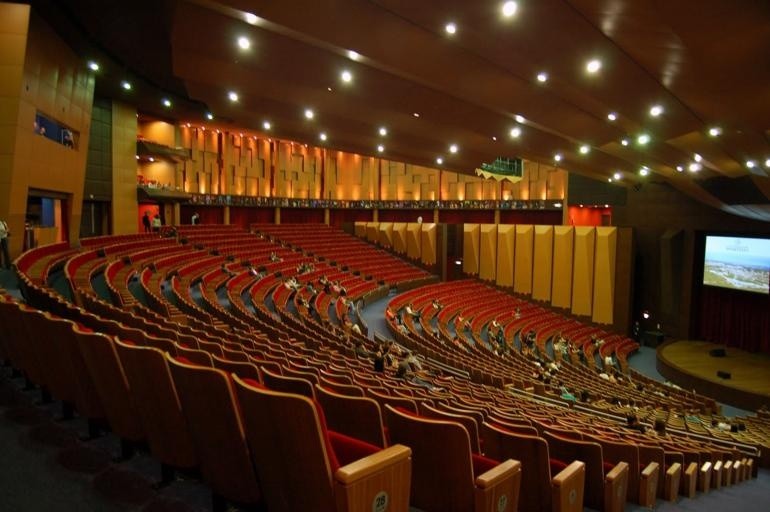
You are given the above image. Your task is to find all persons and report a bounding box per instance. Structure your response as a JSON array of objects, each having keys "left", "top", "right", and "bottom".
[
  {"left": 386, "top": 303, "right": 421, "bottom": 325},
  {"left": 139, "top": 175, "right": 182, "bottom": 190},
  {"left": 248, "top": 251, "right": 362, "bottom": 335},
  {"left": 142, "top": 212, "right": 151, "bottom": 234},
  {"left": 674, "top": 407, "right": 746, "bottom": 433},
  {"left": 620, "top": 413, "right": 646, "bottom": 435},
  {"left": 159, "top": 224, "right": 177, "bottom": 237},
  {"left": 432, "top": 300, "right": 443, "bottom": 309},
  {"left": 192, "top": 212, "right": 200, "bottom": 225},
  {"left": 646, "top": 419, "right": 673, "bottom": 440},
  {"left": 552, "top": 332, "right": 672, "bottom": 406},
  {"left": 0, "top": 216, "right": 10, "bottom": 269},
  {"left": 337, "top": 334, "right": 422, "bottom": 379},
  {"left": 152, "top": 214, "right": 161, "bottom": 236},
  {"left": 433, "top": 307, "right": 562, "bottom": 381}
]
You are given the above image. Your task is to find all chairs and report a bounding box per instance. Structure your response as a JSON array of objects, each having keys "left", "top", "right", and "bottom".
[
  {"left": 442, "top": 277, "right": 640, "bottom": 392},
  {"left": 0, "top": 221, "right": 441, "bottom": 511},
  {"left": 442, "top": 374, "right": 769, "bottom": 512}
]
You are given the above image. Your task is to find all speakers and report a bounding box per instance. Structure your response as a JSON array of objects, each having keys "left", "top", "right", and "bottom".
[
  {"left": 709, "top": 349, "right": 725, "bottom": 356},
  {"left": 717, "top": 371, "right": 730, "bottom": 379}
]
